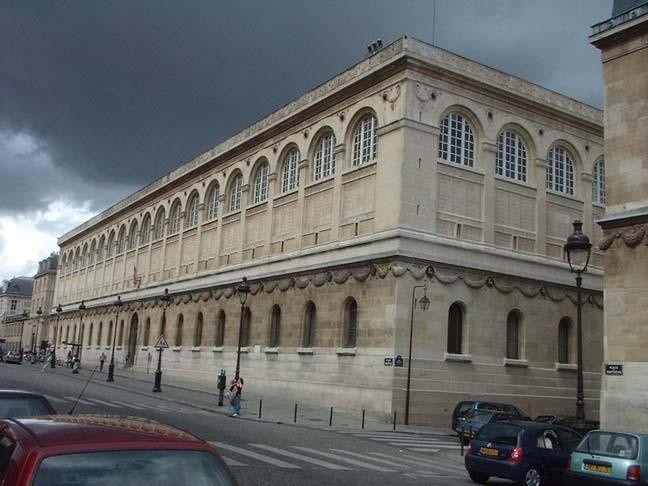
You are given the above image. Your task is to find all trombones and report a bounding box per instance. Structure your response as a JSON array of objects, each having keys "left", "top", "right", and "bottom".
[{"left": 154, "top": 335, "right": 170, "bottom": 348}]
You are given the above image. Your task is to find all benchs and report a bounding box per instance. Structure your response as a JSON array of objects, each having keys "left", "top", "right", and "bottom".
[{"left": 132, "top": 267, "right": 139, "bottom": 289}]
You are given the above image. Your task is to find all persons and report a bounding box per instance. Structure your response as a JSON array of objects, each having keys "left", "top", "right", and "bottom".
[
  {"left": 24, "top": 348, "right": 80, "bottom": 373},
  {"left": 225, "top": 377, "right": 244, "bottom": 417},
  {"left": 217, "top": 369, "right": 227, "bottom": 405},
  {"left": 124, "top": 352, "right": 130, "bottom": 368},
  {"left": 100, "top": 351, "right": 107, "bottom": 373}
]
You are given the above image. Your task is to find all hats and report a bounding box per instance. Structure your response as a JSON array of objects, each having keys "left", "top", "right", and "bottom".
[
  {"left": 452, "top": 401, "right": 648, "bottom": 486},
  {"left": 2, "top": 351, "right": 22, "bottom": 363},
  {"left": 0, "top": 389, "right": 238, "bottom": 486}
]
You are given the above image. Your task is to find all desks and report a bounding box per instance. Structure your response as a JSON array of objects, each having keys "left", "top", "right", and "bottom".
[
  {"left": 152, "top": 288, "right": 170, "bottom": 391},
  {"left": 236, "top": 276, "right": 250, "bottom": 382},
  {"left": 564, "top": 218, "right": 592, "bottom": 431},
  {"left": 75, "top": 300, "right": 87, "bottom": 355},
  {"left": 52, "top": 304, "right": 62, "bottom": 368},
  {"left": 34, "top": 306, "right": 42, "bottom": 352},
  {"left": 405, "top": 280, "right": 430, "bottom": 424},
  {"left": 19, "top": 310, "right": 27, "bottom": 357},
  {"left": 106, "top": 296, "right": 122, "bottom": 382}
]
